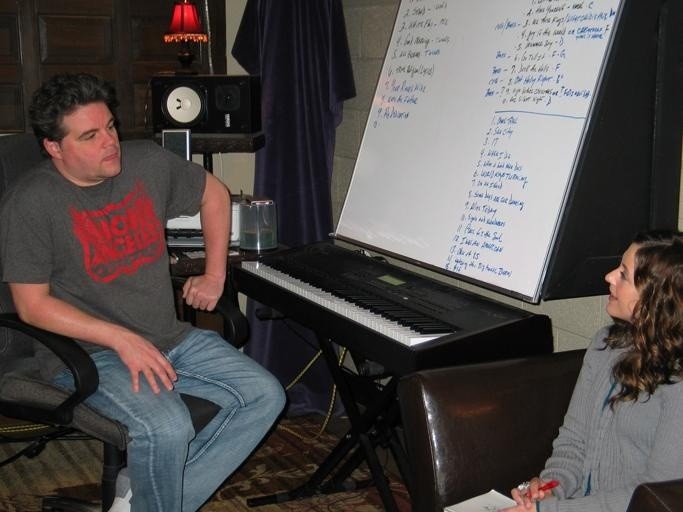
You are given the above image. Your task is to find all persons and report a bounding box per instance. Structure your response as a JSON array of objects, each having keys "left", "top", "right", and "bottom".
[
  {"left": 498, "top": 228, "right": 682, "bottom": 511},
  {"left": 0, "top": 75, "right": 288, "bottom": 511}
]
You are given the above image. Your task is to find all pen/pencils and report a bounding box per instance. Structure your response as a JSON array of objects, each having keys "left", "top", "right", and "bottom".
[{"left": 527, "top": 480, "right": 560, "bottom": 497}]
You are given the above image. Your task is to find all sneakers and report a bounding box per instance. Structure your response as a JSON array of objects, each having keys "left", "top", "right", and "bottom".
[{"left": 106, "top": 467, "right": 132, "bottom": 512}]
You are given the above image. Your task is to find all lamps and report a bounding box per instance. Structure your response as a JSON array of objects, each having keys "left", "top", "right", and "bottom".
[{"left": 163, "top": 0, "right": 208, "bottom": 74}]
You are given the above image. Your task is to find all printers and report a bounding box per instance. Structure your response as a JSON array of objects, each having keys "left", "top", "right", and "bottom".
[{"left": 165, "top": 197, "right": 244, "bottom": 250}]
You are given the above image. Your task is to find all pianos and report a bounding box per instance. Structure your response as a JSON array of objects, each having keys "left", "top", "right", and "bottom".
[{"left": 231, "top": 241, "right": 554, "bottom": 374}]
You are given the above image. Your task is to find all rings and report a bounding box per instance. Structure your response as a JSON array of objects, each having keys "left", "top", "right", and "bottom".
[{"left": 515, "top": 479, "right": 531, "bottom": 493}]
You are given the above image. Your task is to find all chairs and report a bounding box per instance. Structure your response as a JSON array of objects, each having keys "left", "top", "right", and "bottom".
[
  {"left": 0, "top": 134, "right": 250, "bottom": 512},
  {"left": 398, "top": 348, "right": 682, "bottom": 512}
]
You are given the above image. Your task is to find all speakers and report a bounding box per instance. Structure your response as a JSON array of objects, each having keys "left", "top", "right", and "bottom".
[{"left": 151, "top": 76, "right": 262, "bottom": 134}]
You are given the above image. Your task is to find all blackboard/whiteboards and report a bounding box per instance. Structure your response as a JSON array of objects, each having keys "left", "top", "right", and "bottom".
[{"left": 332, "top": 0, "right": 628, "bottom": 306}]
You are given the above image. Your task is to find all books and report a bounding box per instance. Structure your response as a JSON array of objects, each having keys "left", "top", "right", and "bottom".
[{"left": 443, "top": 488, "right": 518, "bottom": 512}]
